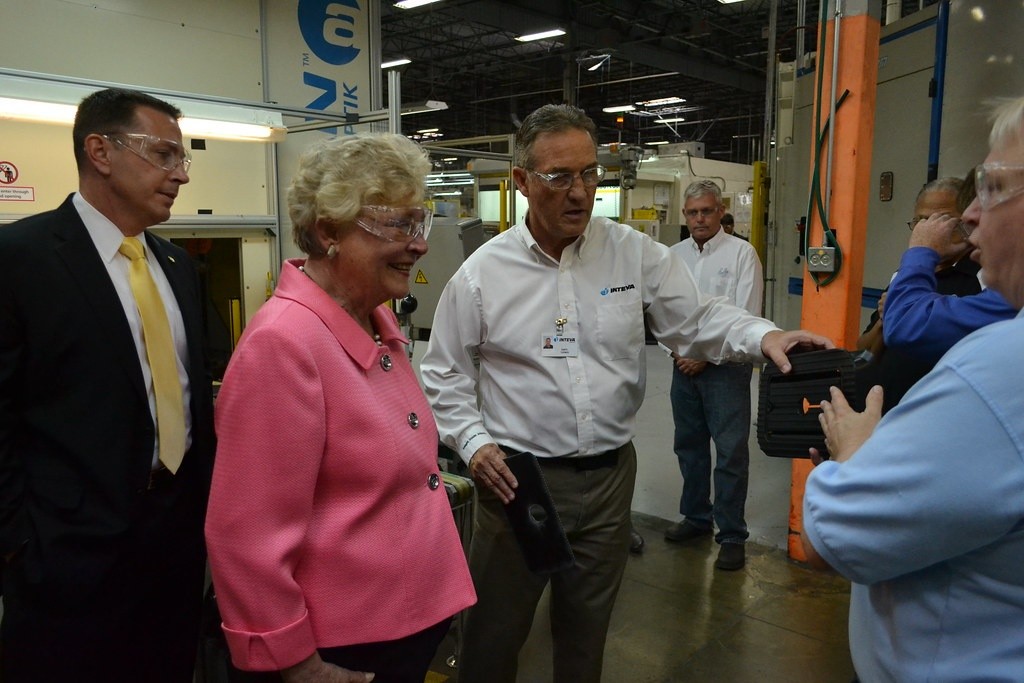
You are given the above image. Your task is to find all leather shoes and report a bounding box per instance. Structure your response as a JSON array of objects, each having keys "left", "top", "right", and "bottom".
[{"left": 630, "top": 528, "right": 644, "bottom": 551}]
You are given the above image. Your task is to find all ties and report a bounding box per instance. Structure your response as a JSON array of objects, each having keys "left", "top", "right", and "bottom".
[{"left": 120, "top": 235, "right": 185, "bottom": 474}]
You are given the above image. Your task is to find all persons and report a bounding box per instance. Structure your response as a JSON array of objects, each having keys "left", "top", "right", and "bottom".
[
  {"left": 852, "top": 161, "right": 1020, "bottom": 379},
  {"left": 650, "top": 179, "right": 765, "bottom": 570},
  {"left": 203, "top": 127, "right": 480, "bottom": 683},
  {"left": 543, "top": 338, "right": 553, "bottom": 350},
  {"left": 801, "top": 94, "right": 1024, "bottom": 683},
  {"left": 421, "top": 100, "right": 835, "bottom": 682},
  {"left": 0, "top": 88, "right": 216, "bottom": 683}
]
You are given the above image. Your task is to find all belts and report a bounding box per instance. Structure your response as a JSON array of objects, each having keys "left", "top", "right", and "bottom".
[{"left": 538, "top": 449, "right": 618, "bottom": 470}]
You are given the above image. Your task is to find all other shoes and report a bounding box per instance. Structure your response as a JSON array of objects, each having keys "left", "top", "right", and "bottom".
[
  {"left": 664, "top": 519, "right": 714, "bottom": 541},
  {"left": 714, "top": 543, "right": 745, "bottom": 570}
]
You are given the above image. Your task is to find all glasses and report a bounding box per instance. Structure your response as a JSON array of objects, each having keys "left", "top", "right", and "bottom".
[
  {"left": 974, "top": 161, "right": 1024, "bottom": 210},
  {"left": 525, "top": 164, "right": 605, "bottom": 191},
  {"left": 82, "top": 133, "right": 193, "bottom": 170},
  {"left": 356, "top": 207, "right": 434, "bottom": 243},
  {"left": 907, "top": 221, "right": 918, "bottom": 231}
]
